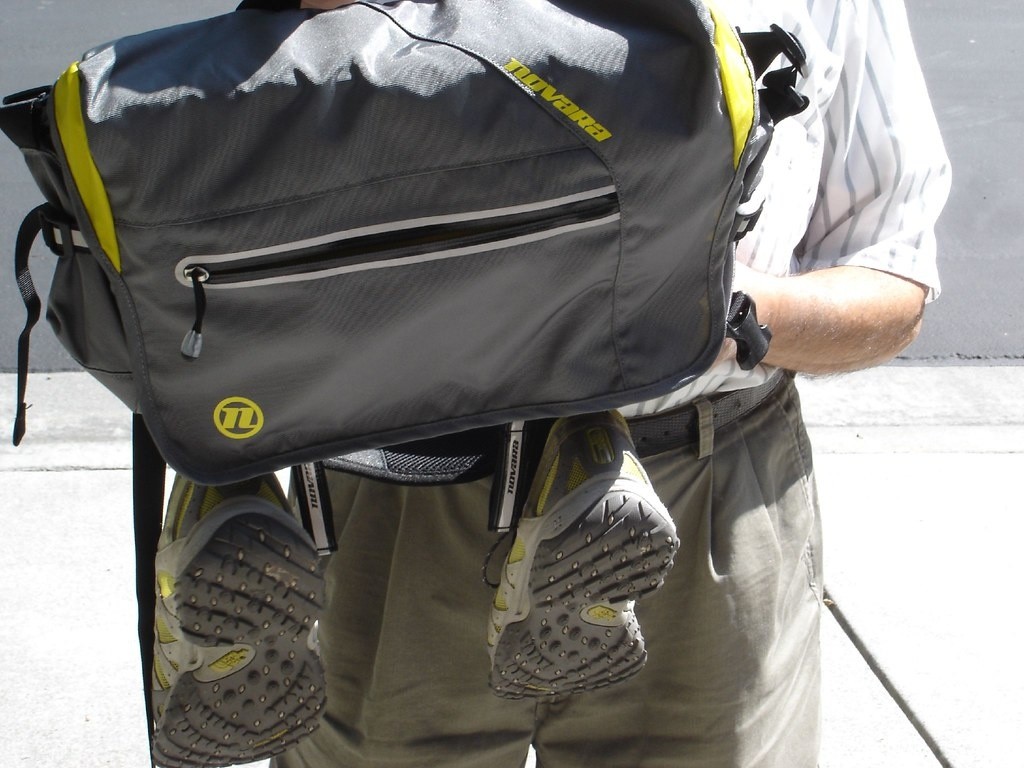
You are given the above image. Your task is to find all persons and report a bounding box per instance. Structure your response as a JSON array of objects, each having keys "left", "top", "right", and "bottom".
[{"left": 265, "top": 0, "right": 953, "bottom": 768}]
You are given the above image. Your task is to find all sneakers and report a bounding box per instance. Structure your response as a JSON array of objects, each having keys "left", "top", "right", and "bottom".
[
  {"left": 482, "top": 408, "right": 680, "bottom": 699},
  {"left": 148, "top": 467, "right": 328, "bottom": 768}
]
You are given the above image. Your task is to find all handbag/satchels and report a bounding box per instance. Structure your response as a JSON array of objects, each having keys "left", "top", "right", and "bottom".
[{"left": 1, "top": 0, "right": 810, "bottom": 487}]
